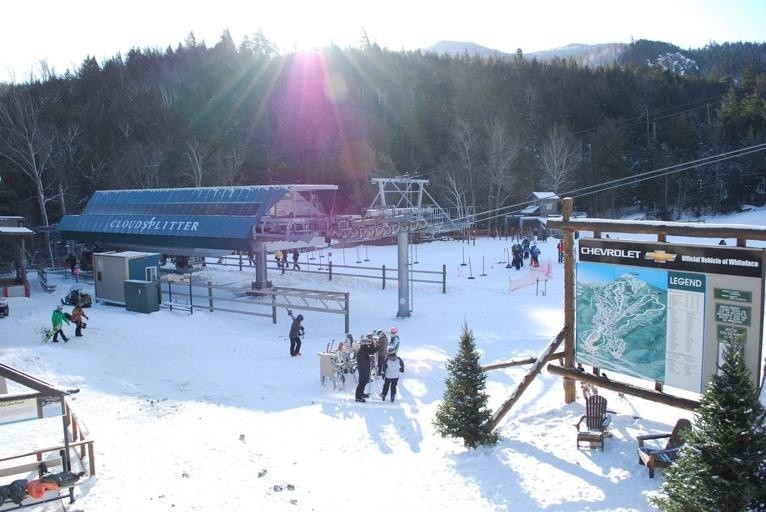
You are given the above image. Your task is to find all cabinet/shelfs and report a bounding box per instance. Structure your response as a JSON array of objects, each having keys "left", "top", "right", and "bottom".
[{"left": 123, "top": 279, "right": 160, "bottom": 313}]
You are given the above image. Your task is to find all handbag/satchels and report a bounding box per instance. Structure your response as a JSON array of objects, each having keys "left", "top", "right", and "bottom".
[{"left": 0, "top": 472, "right": 80, "bottom": 506}]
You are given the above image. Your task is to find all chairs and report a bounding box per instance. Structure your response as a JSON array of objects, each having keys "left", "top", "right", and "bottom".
[
  {"left": 574, "top": 395, "right": 611, "bottom": 451},
  {"left": 636, "top": 419, "right": 692, "bottom": 478}
]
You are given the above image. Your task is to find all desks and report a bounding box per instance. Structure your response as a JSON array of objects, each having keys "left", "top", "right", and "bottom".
[{"left": 316, "top": 342, "right": 361, "bottom": 389}]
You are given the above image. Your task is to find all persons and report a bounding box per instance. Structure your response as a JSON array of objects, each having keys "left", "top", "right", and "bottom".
[
  {"left": 490, "top": 224, "right": 564, "bottom": 270},
  {"left": 245, "top": 248, "right": 303, "bottom": 270},
  {"left": 52, "top": 304, "right": 70, "bottom": 342},
  {"left": 73, "top": 264, "right": 81, "bottom": 283},
  {"left": 355, "top": 328, "right": 405, "bottom": 402},
  {"left": 287, "top": 313, "right": 305, "bottom": 357},
  {"left": 74, "top": 305, "right": 88, "bottom": 337}
]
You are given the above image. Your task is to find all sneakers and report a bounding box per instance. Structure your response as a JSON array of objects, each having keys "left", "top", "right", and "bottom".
[{"left": 356, "top": 393, "right": 385, "bottom": 402}]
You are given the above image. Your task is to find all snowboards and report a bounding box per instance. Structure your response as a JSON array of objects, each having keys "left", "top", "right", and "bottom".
[
  {"left": 64, "top": 312, "right": 86, "bottom": 329},
  {"left": 42, "top": 325, "right": 60, "bottom": 343}
]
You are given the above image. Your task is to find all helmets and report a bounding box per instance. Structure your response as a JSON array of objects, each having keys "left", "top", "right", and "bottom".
[
  {"left": 297, "top": 314, "right": 304, "bottom": 322},
  {"left": 361, "top": 327, "right": 398, "bottom": 355}
]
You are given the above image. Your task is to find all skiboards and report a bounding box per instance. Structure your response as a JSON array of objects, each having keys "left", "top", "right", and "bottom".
[{"left": 327, "top": 330, "right": 381, "bottom": 391}]
